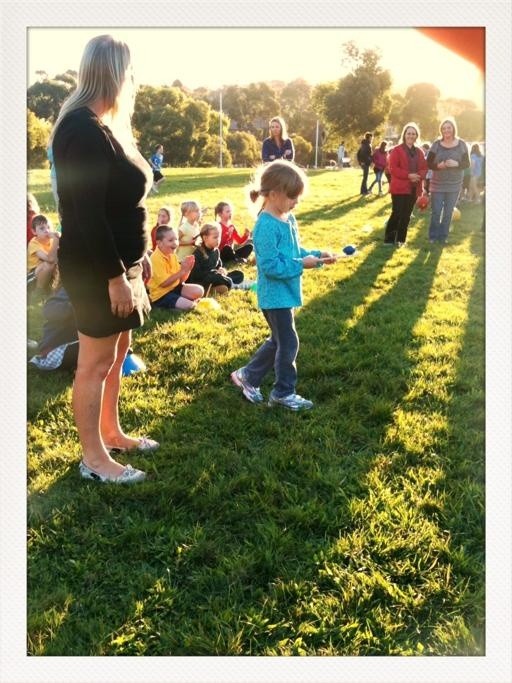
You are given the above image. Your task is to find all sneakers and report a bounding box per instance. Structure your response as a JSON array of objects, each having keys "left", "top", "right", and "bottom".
[
  {"left": 230, "top": 367, "right": 263, "bottom": 405},
  {"left": 105, "top": 434, "right": 160, "bottom": 454},
  {"left": 267, "top": 391, "right": 314, "bottom": 413},
  {"left": 77, "top": 461, "right": 148, "bottom": 485}
]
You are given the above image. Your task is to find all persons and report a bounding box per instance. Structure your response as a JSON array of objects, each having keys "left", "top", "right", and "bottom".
[
  {"left": 420, "top": 144, "right": 432, "bottom": 198},
  {"left": 426, "top": 120, "right": 470, "bottom": 245},
  {"left": 146, "top": 224, "right": 205, "bottom": 312},
  {"left": 337, "top": 141, "right": 347, "bottom": 171},
  {"left": 215, "top": 202, "right": 253, "bottom": 266},
  {"left": 27, "top": 216, "right": 61, "bottom": 302},
  {"left": 262, "top": 115, "right": 294, "bottom": 162},
  {"left": 384, "top": 123, "right": 428, "bottom": 247},
  {"left": 368, "top": 141, "right": 387, "bottom": 194},
  {"left": 356, "top": 134, "right": 370, "bottom": 195},
  {"left": 46, "top": 35, "right": 160, "bottom": 485},
  {"left": 178, "top": 201, "right": 203, "bottom": 261},
  {"left": 147, "top": 143, "right": 166, "bottom": 195},
  {"left": 29, "top": 288, "right": 133, "bottom": 374},
  {"left": 28, "top": 195, "right": 39, "bottom": 245},
  {"left": 461, "top": 144, "right": 484, "bottom": 204},
  {"left": 151, "top": 208, "right": 171, "bottom": 251},
  {"left": 231, "top": 163, "right": 337, "bottom": 411},
  {"left": 186, "top": 224, "right": 232, "bottom": 295}
]
